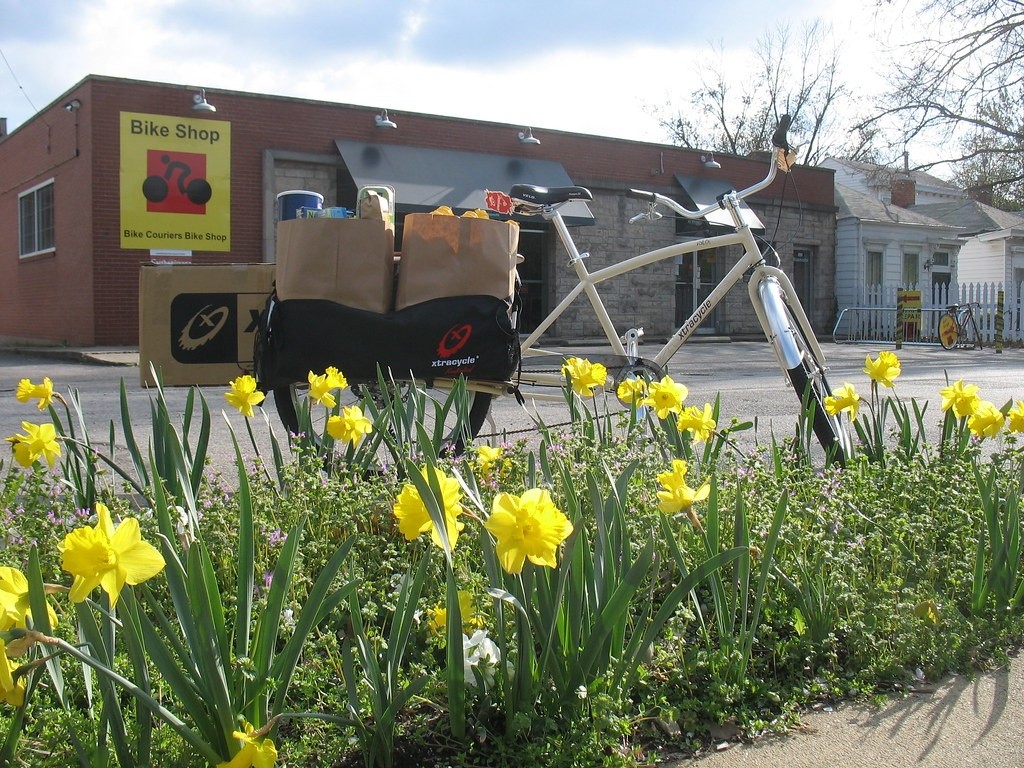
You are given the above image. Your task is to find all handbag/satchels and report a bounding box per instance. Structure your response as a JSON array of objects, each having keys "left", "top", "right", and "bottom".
[{"left": 249, "top": 210, "right": 524, "bottom": 383}]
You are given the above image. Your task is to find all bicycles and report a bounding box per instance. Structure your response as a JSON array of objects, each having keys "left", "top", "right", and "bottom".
[
  {"left": 249, "top": 112, "right": 858, "bottom": 483},
  {"left": 937, "top": 302, "right": 984, "bottom": 351}
]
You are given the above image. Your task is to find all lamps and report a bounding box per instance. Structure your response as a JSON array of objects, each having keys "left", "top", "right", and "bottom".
[
  {"left": 700, "top": 151, "right": 721, "bottom": 169},
  {"left": 375, "top": 109, "right": 397, "bottom": 129},
  {"left": 518, "top": 127, "right": 541, "bottom": 145},
  {"left": 191, "top": 89, "right": 217, "bottom": 113}
]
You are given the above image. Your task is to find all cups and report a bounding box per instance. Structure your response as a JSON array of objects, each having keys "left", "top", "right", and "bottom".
[{"left": 277, "top": 190, "right": 324, "bottom": 221}]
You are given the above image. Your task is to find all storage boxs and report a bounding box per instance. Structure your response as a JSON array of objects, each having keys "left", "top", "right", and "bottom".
[
  {"left": 275, "top": 218, "right": 395, "bottom": 312},
  {"left": 396, "top": 212, "right": 520, "bottom": 330},
  {"left": 137, "top": 260, "right": 276, "bottom": 388}
]
